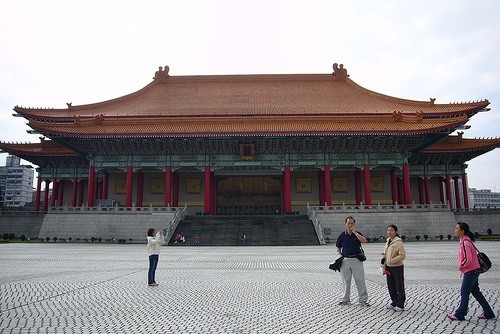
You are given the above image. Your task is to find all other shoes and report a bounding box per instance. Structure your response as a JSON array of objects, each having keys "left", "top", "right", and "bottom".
[
  {"left": 386, "top": 304, "right": 394, "bottom": 309},
  {"left": 361, "top": 302, "right": 371, "bottom": 307},
  {"left": 340, "top": 298, "right": 350, "bottom": 303},
  {"left": 477, "top": 312, "right": 487, "bottom": 319},
  {"left": 448, "top": 314, "right": 458, "bottom": 320},
  {"left": 148, "top": 282, "right": 159, "bottom": 286},
  {"left": 393, "top": 306, "right": 404, "bottom": 311}
]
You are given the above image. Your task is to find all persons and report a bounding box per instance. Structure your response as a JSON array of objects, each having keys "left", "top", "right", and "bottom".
[
  {"left": 195, "top": 234, "right": 200, "bottom": 245},
  {"left": 335, "top": 216, "right": 371, "bottom": 306},
  {"left": 173, "top": 231, "right": 186, "bottom": 244},
  {"left": 381, "top": 225, "right": 406, "bottom": 311},
  {"left": 241, "top": 232, "right": 246, "bottom": 244},
  {"left": 146, "top": 228, "right": 164, "bottom": 286}
]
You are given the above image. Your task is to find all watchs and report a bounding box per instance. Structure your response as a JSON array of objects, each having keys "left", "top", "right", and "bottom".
[{"left": 447, "top": 222, "right": 496, "bottom": 321}]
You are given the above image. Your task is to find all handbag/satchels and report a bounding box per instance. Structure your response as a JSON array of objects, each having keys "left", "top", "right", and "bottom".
[
  {"left": 355, "top": 250, "right": 366, "bottom": 262},
  {"left": 463, "top": 239, "right": 492, "bottom": 273}
]
